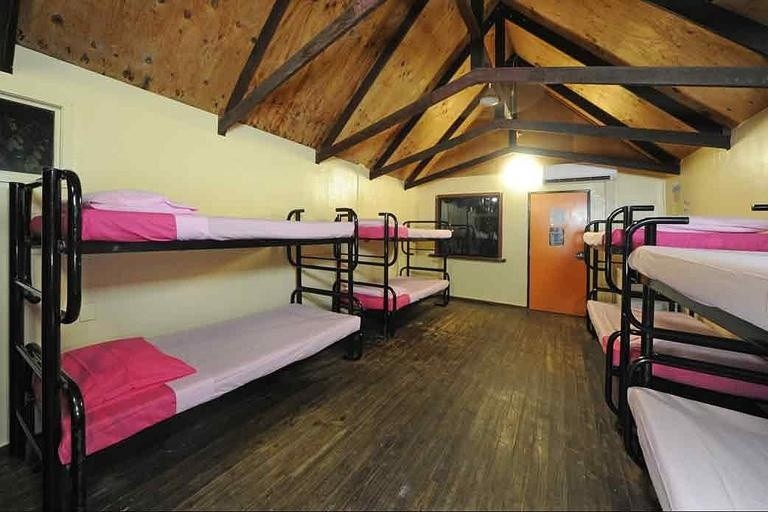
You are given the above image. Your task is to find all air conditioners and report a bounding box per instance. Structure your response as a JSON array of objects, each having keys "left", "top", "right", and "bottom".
[{"left": 544, "top": 163, "right": 617, "bottom": 183}]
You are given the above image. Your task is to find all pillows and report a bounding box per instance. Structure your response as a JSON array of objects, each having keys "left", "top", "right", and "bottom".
[
  {"left": 345, "top": 282, "right": 408, "bottom": 298},
  {"left": 62, "top": 188, "right": 196, "bottom": 214}
]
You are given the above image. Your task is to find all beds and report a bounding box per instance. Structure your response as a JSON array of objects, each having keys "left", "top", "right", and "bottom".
[
  {"left": 339, "top": 266, "right": 451, "bottom": 335},
  {"left": 356, "top": 219, "right": 454, "bottom": 242},
  {"left": 575, "top": 201, "right": 768, "bottom": 511},
  {"left": 8, "top": 168, "right": 358, "bottom": 254},
  {"left": 25, "top": 285, "right": 366, "bottom": 510}
]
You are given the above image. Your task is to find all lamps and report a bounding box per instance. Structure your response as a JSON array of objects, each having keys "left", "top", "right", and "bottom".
[{"left": 480, "top": 85, "right": 499, "bottom": 106}]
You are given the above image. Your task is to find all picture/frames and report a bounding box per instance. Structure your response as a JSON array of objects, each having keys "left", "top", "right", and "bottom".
[{"left": 0, "top": 88, "right": 63, "bottom": 186}]
